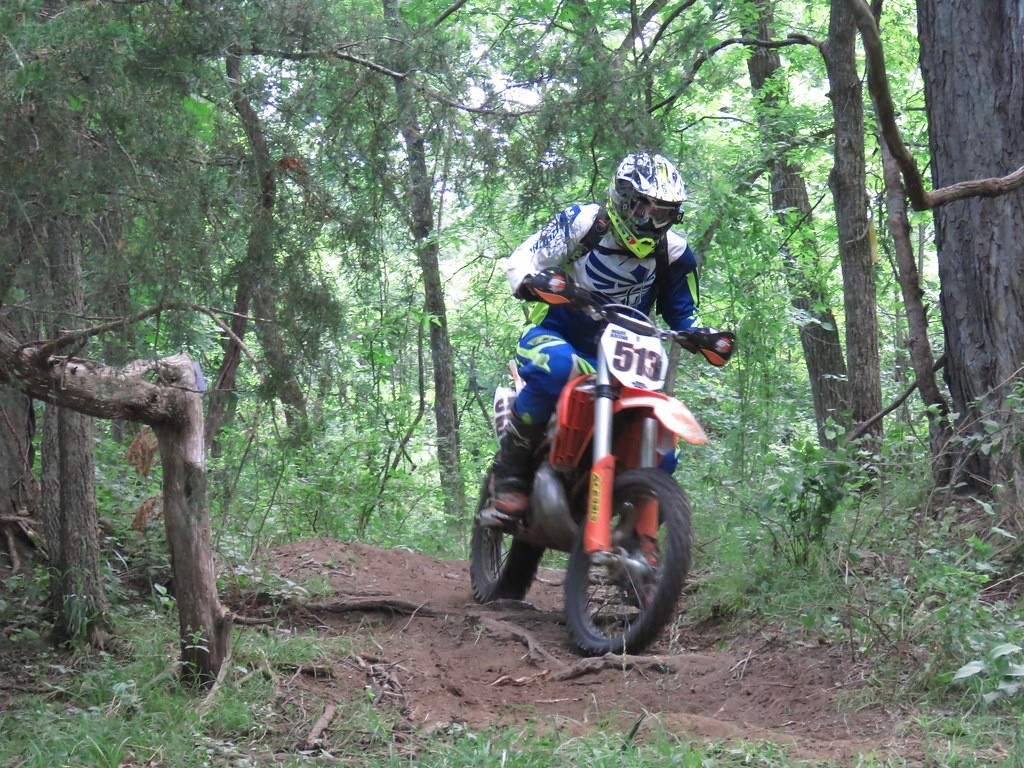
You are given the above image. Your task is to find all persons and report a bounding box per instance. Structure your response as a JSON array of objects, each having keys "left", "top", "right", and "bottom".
[{"left": 485, "top": 150, "right": 734, "bottom": 518}]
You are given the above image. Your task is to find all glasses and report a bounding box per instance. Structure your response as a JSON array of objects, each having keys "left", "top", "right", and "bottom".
[{"left": 608, "top": 182, "right": 684, "bottom": 231}]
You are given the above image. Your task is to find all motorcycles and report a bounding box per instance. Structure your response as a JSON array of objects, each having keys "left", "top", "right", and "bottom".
[{"left": 468, "top": 275, "right": 737, "bottom": 658}]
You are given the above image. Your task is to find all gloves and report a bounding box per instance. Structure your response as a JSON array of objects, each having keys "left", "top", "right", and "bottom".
[
  {"left": 694, "top": 326, "right": 736, "bottom": 367},
  {"left": 533, "top": 267, "right": 576, "bottom": 303}
]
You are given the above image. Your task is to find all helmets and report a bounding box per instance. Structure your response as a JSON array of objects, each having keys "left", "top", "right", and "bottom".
[{"left": 608, "top": 153, "right": 688, "bottom": 259}]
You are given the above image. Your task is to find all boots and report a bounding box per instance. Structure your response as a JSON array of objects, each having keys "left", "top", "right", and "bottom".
[{"left": 486, "top": 402, "right": 550, "bottom": 516}]
